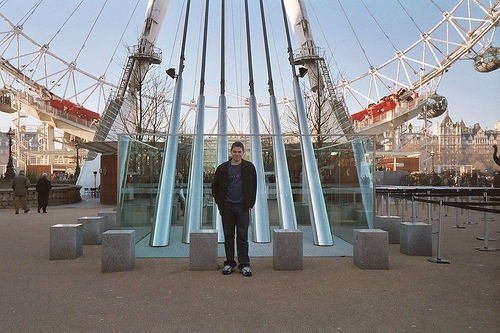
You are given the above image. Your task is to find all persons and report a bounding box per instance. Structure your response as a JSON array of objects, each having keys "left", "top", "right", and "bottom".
[
  {"left": 52, "top": 172, "right": 74, "bottom": 181},
  {"left": 448, "top": 173, "right": 500, "bottom": 187},
  {"left": 212, "top": 141, "right": 257, "bottom": 277},
  {"left": 12, "top": 169, "right": 30, "bottom": 214},
  {"left": 432, "top": 171, "right": 437, "bottom": 184},
  {"left": 36, "top": 173, "right": 51, "bottom": 213},
  {"left": 176, "top": 170, "right": 188, "bottom": 216}
]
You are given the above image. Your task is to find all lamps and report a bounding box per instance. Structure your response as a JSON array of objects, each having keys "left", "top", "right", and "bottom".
[
  {"left": 297, "top": 68, "right": 308, "bottom": 78},
  {"left": 166, "top": 68, "right": 178, "bottom": 79}
]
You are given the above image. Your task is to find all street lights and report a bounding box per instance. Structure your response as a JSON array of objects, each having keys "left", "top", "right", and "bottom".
[
  {"left": 74, "top": 140, "right": 80, "bottom": 185},
  {"left": 3, "top": 126, "right": 16, "bottom": 180}
]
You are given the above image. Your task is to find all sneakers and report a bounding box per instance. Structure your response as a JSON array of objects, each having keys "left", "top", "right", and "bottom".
[
  {"left": 222, "top": 266, "right": 235, "bottom": 274},
  {"left": 241, "top": 267, "right": 252, "bottom": 276}
]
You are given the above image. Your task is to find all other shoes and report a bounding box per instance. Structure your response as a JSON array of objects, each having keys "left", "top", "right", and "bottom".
[
  {"left": 24, "top": 209, "right": 30, "bottom": 213},
  {"left": 16, "top": 211, "right": 19, "bottom": 214},
  {"left": 43, "top": 212, "right": 47, "bottom": 213},
  {"left": 38, "top": 208, "right": 40, "bottom": 213}
]
